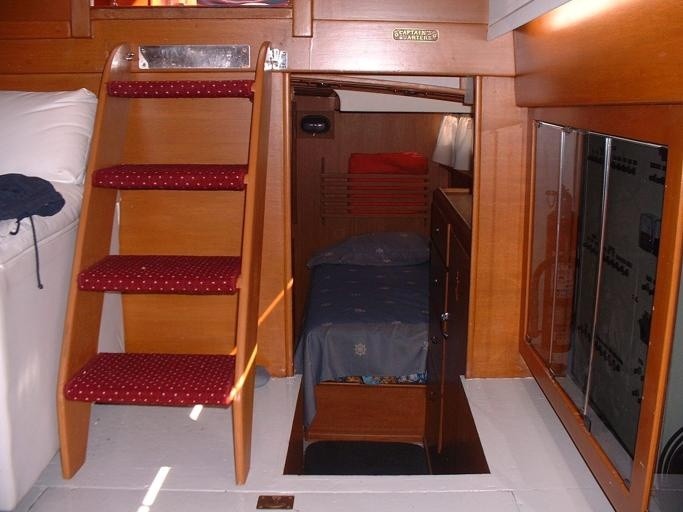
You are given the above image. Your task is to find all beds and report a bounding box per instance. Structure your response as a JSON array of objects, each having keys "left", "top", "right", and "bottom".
[
  {"left": 304, "top": 263, "right": 428, "bottom": 442},
  {"left": 2, "top": 184, "right": 130, "bottom": 509}
]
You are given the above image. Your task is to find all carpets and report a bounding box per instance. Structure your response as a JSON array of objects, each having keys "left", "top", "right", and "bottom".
[{"left": 306, "top": 440, "right": 427, "bottom": 475}]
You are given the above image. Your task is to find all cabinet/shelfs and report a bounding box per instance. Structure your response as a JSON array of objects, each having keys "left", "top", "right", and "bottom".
[{"left": 426, "top": 185, "right": 472, "bottom": 450}]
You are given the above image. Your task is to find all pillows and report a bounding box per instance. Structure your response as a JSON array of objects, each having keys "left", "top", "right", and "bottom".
[
  {"left": 331, "top": 234, "right": 429, "bottom": 265},
  {"left": 0, "top": 84, "right": 98, "bottom": 181}
]
